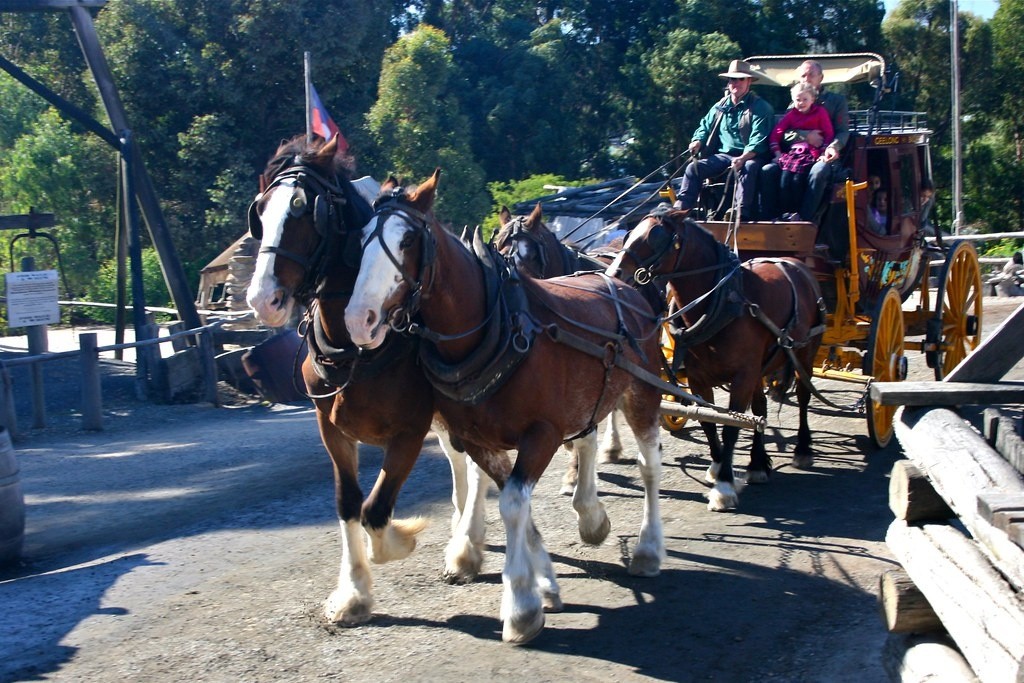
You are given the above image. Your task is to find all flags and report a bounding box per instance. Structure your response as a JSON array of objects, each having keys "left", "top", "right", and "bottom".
[{"left": 309, "top": 83, "right": 349, "bottom": 152}]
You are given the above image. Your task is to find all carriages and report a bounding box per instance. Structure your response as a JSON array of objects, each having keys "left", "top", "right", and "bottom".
[{"left": 247, "top": 53, "right": 984, "bottom": 648}]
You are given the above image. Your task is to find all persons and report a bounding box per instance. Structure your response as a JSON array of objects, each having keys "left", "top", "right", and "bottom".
[
  {"left": 753, "top": 60, "right": 849, "bottom": 221},
  {"left": 867, "top": 174, "right": 888, "bottom": 235},
  {"left": 920, "top": 180, "right": 933, "bottom": 206},
  {"left": 671, "top": 60, "right": 774, "bottom": 222}
]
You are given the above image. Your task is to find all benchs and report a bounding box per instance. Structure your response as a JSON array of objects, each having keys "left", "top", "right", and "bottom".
[{"left": 700, "top": 131, "right": 857, "bottom": 222}]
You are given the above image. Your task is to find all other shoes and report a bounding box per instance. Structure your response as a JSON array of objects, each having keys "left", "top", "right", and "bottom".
[
  {"left": 724, "top": 207, "right": 753, "bottom": 221},
  {"left": 764, "top": 205, "right": 782, "bottom": 219},
  {"left": 772, "top": 212, "right": 802, "bottom": 221},
  {"left": 659, "top": 200, "right": 692, "bottom": 220},
  {"left": 815, "top": 242, "right": 828, "bottom": 250}
]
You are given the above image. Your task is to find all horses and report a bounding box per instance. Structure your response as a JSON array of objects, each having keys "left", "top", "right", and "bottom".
[
  {"left": 343, "top": 166, "right": 669, "bottom": 646},
  {"left": 489, "top": 198, "right": 634, "bottom": 502},
  {"left": 243, "top": 127, "right": 490, "bottom": 632},
  {"left": 604, "top": 200, "right": 825, "bottom": 513}
]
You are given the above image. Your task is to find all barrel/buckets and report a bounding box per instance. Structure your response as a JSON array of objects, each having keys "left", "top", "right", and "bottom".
[
  {"left": 241, "top": 329, "right": 308, "bottom": 402},
  {"left": 0, "top": 425, "right": 26, "bottom": 564}
]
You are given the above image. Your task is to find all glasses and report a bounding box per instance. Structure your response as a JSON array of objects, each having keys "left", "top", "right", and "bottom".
[{"left": 730, "top": 77, "right": 746, "bottom": 82}]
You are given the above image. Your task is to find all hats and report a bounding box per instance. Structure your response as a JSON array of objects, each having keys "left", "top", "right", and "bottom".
[{"left": 718, "top": 60, "right": 759, "bottom": 82}]
